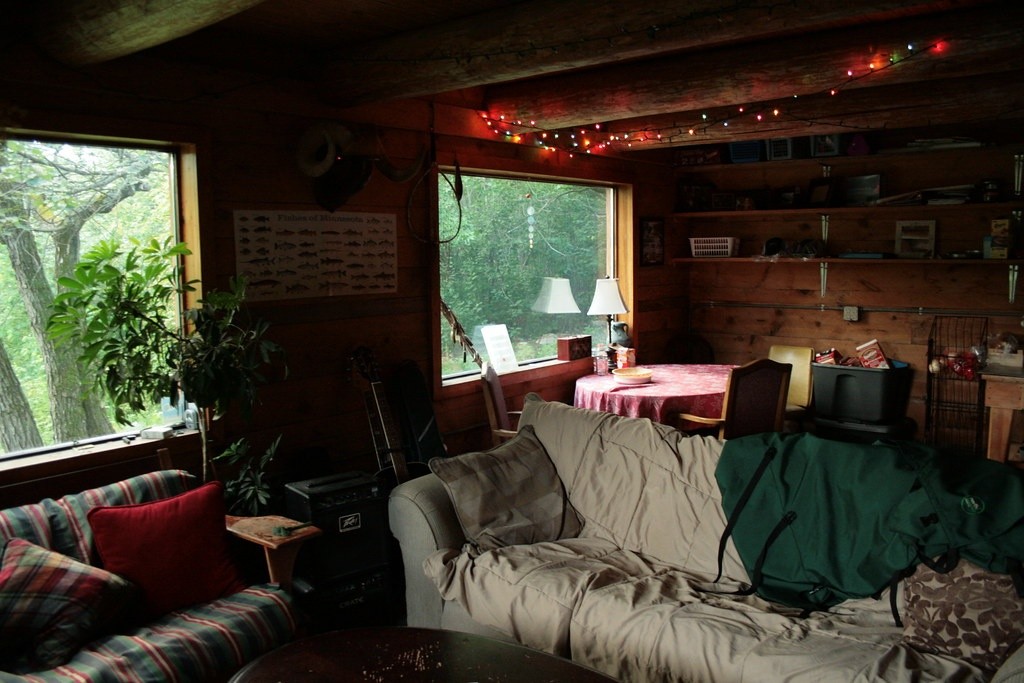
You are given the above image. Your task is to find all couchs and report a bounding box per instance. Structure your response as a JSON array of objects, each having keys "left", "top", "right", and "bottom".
[{"left": 388, "top": 395, "right": 1024, "bottom": 683}]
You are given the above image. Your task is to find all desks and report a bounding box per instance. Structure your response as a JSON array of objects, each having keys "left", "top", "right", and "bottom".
[
  {"left": 980, "top": 375, "right": 1024, "bottom": 461},
  {"left": 573, "top": 362, "right": 742, "bottom": 429}
]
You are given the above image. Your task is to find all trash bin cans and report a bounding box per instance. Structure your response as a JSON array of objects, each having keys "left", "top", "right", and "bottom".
[{"left": 283, "top": 471, "right": 386, "bottom": 629}]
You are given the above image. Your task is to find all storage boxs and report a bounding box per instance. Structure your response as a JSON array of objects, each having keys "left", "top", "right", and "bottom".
[
  {"left": 809, "top": 357, "right": 911, "bottom": 420},
  {"left": 816, "top": 418, "right": 916, "bottom": 446},
  {"left": 688, "top": 236, "right": 740, "bottom": 258}
]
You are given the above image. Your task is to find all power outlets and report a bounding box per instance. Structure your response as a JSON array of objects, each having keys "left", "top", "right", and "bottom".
[{"left": 844, "top": 307, "right": 858, "bottom": 321}]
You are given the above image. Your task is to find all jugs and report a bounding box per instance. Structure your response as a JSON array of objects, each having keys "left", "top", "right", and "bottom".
[{"left": 607, "top": 321, "right": 631, "bottom": 347}]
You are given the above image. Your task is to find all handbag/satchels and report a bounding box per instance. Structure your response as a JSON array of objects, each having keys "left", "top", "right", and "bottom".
[{"left": 715, "top": 430, "right": 1024, "bottom": 615}]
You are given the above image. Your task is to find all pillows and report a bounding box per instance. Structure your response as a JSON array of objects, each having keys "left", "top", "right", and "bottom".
[
  {"left": 85, "top": 478, "right": 247, "bottom": 615},
  {"left": 1, "top": 533, "right": 133, "bottom": 674},
  {"left": 897, "top": 555, "right": 1024, "bottom": 672},
  {"left": 427, "top": 426, "right": 586, "bottom": 557}
]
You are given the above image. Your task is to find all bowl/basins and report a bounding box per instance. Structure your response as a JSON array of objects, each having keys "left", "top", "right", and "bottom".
[{"left": 611, "top": 368, "right": 653, "bottom": 384}]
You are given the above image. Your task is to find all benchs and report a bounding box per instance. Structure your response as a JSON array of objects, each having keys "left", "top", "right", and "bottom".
[{"left": 0, "top": 468, "right": 321, "bottom": 682}]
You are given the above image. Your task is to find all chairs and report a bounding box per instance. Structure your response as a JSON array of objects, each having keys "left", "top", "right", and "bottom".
[
  {"left": 668, "top": 355, "right": 793, "bottom": 442},
  {"left": 768, "top": 344, "right": 815, "bottom": 438}
]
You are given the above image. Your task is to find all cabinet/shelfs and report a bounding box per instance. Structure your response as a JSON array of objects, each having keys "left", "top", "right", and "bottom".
[{"left": 666, "top": 157, "right": 1024, "bottom": 264}]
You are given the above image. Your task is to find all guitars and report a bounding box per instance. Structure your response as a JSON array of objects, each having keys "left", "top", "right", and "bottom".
[{"left": 346, "top": 346, "right": 432, "bottom": 498}]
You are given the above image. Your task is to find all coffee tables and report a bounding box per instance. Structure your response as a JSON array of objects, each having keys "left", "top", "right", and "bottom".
[{"left": 227, "top": 626, "right": 619, "bottom": 683}]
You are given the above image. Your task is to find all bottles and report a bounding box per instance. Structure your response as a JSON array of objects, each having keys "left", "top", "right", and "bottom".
[{"left": 594, "top": 343, "right": 608, "bottom": 377}]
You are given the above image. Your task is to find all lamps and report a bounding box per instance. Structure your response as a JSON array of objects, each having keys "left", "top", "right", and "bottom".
[
  {"left": 531, "top": 277, "right": 582, "bottom": 315},
  {"left": 586, "top": 276, "right": 629, "bottom": 372}
]
